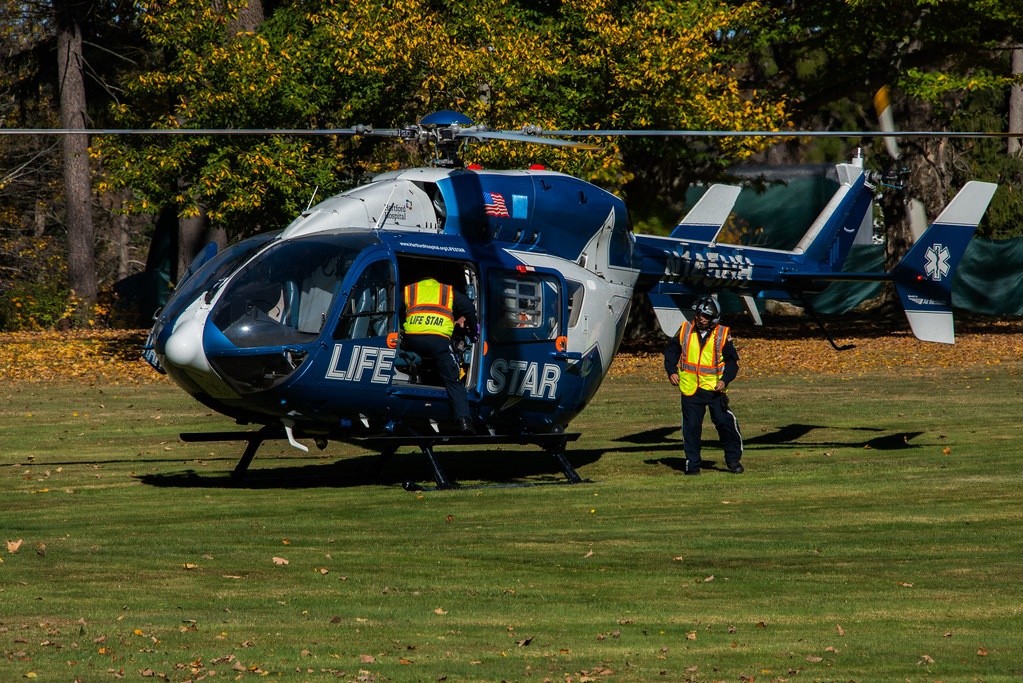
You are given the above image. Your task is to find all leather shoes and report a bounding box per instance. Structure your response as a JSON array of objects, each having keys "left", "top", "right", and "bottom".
[
  {"left": 409, "top": 375, "right": 422, "bottom": 383},
  {"left": 458, "top": 416, "right": 476, "bottom": 435}
]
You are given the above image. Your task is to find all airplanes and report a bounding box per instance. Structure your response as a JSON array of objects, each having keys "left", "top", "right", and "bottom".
[{"left": 0, "top": 108, "right": 1022, "bottom": 492}]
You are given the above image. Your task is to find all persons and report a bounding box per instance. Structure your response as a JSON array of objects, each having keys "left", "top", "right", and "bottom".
[
  {"left": 401, "top": 278, "right": 475, "bottom": 433},
  {"left": 664, "top": 297, "right": 744, "bottom": 475}
]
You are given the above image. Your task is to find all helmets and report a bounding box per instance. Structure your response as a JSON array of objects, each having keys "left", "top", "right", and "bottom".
[{"left": 692, "top": 296, "right": 720, "bottom": 319}]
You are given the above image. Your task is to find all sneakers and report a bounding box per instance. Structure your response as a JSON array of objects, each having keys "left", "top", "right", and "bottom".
[
  {"left": 685, "top": 470, "right": 700, "bottom": 475},
  {"left": 727, "top": 462, "right": 744, "bottom": 473}
]
